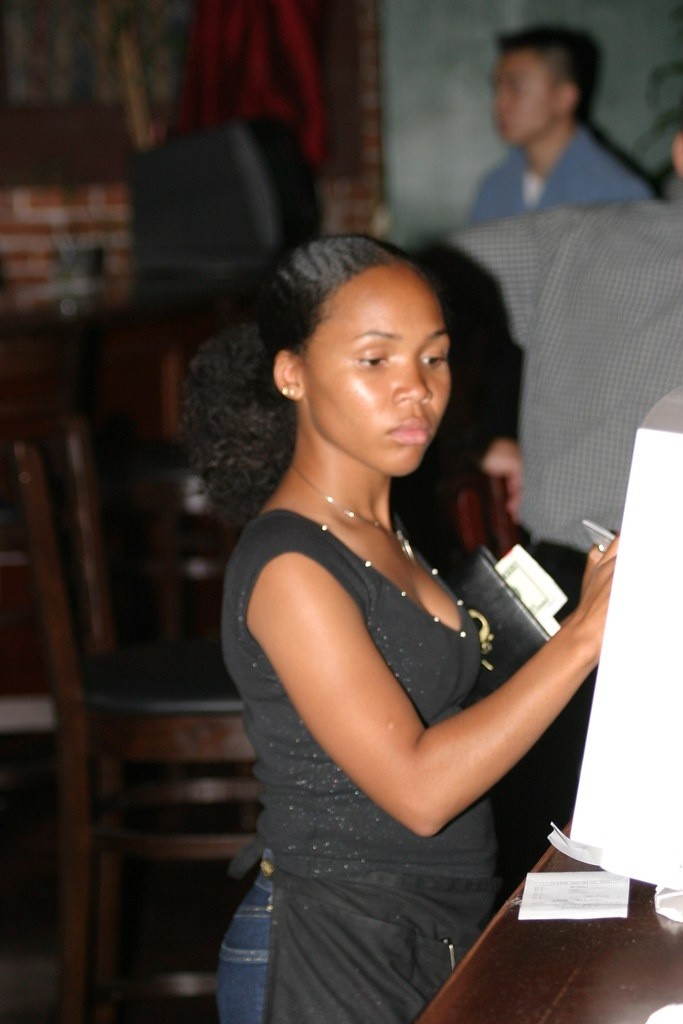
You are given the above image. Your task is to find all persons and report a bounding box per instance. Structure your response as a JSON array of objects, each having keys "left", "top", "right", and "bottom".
[
  {"left": 416, "top": 23, "right": 683, "bottom": 817},
  {"left": 216, "top": 235, "right": 622, "bottom": 1024}
]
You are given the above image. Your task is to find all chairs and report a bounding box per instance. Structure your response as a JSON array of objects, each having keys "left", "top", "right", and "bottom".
[{"left": 11, "top": 340, "right": 259, "bottom": 1024}]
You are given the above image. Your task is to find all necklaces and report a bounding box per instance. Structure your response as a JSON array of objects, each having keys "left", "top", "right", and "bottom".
[{"left": 288, "top": 462, "right": 415, "bottom": 562}]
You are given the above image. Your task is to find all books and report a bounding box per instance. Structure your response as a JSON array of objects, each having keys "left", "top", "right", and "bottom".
[{"left": 460, "top": 543, "right": 568, "bottom": 694}]
